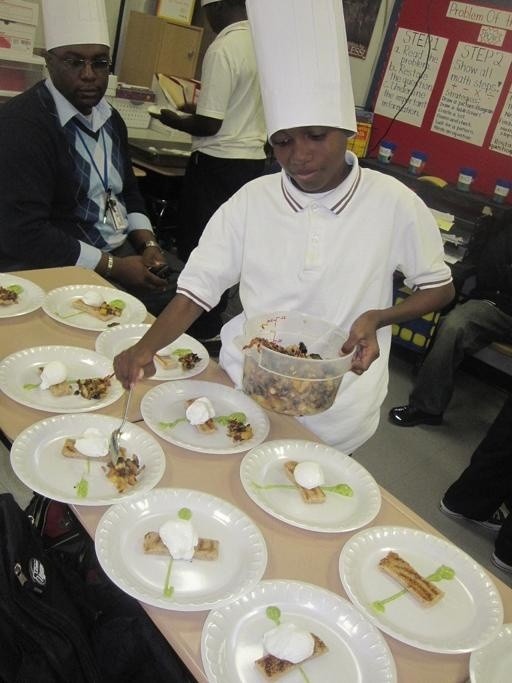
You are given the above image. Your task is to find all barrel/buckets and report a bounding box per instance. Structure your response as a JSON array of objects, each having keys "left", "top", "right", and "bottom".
[{"left": 234, "top": 312, "right": 366, "bottom": 419}]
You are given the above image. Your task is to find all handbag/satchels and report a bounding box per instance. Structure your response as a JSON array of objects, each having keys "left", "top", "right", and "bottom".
[{"left": 0, "top": 492, "right": 195, "bottom": 682}]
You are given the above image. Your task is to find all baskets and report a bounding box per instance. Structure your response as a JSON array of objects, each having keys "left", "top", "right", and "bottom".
[{"left": 104, "top": 75, "right": 157, "bottom": 129}]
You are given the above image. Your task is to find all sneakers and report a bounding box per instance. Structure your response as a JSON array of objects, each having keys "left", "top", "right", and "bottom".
[{"left": 440, "top": 493, "right": 512, "bottom": 574}]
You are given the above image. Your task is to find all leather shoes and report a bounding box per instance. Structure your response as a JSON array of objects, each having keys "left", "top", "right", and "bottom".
[{"left": 388, "top": 404, "right": 443, "bottom": 426}]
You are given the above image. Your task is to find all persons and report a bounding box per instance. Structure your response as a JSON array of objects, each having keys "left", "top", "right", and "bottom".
[
  {"left": 440, "top": 393, "right": 512, "bottom": 573},
  {"left": 151, "top": 0, "right": 270, "bottom": 345},
  {"left": 112, "top": 2, "right": 457, "bottom": 457},
  {"left": 389, "top": 190, "right": 512, "bottom": 428},
  {"left": 1, "top": 0, "right": 170, "bottom": 297}
]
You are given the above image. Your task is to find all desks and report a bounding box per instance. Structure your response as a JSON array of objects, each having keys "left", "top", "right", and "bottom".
[{"left": 0, "top": 266, "right": 512, "bottom": 683}]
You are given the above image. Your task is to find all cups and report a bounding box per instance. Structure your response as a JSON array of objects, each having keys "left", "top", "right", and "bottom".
[{"left": 377, "top": 140, "right": 512, "bottom": 204}]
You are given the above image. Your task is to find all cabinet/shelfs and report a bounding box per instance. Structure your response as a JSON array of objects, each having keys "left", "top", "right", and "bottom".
[{"left": 119, "top": 9, "right": 204, "bottom": 89}]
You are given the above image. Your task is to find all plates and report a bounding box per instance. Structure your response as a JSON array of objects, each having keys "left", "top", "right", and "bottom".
[
  {"left": 337, "top": 526, "right": 504, "bottom": 656},
  {"left": 1, "top": 345, "right": 128, "bottom": 413},
  {"left": 200, "top": 580, "right": 398, "bottom": 682},
  {"left": 94, "top": 489, "right": 269, "bottom": 610},
  {"left": 469, "top": 622, "right": 510, "bottom": 680},
  {"left": 147, "top": 105, "right": 193, "bottom": 120},
  {"left": 241, "top": 440, "right": 383, "bottom": 536},
  {"left": 94, "top": 322, "right": 210, "bottom": 382},
  {"left": 0, "top": 274, "right": 45, "bottom": 320},
  {"left": 9, "top": 413, "right": 164, "bottom": 511},
  {"left": 42, "top": 284, "right": 148, "bottom": 334},
  {"left": 141, "top": 382, "right": 269, "bottom": 456}
]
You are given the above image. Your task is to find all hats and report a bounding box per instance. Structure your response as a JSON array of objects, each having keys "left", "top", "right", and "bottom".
[
  {"left": 41, "top": 0, "right": 111, "bottom": 51},
  {"left": 245, "top": 0, "right": 359, "bottom": 145}
]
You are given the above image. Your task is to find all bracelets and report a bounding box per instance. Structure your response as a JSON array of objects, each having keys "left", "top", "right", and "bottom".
[
  {"left": 104, "top": 253, "right": 114, "bottom": 279},
  {"left": 143, "top": 241, "right": 168, "bottom": 257}
]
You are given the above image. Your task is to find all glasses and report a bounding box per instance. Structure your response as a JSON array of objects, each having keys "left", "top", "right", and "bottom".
[{"left": 48, "top": 51, "right": 112, "bottom": 70}]
